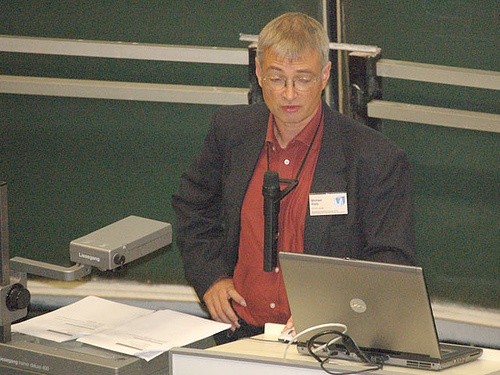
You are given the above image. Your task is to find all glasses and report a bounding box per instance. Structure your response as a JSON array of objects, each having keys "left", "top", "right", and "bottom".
[{"left": 260, "top": 67, "right": 323, "bottom": 91}]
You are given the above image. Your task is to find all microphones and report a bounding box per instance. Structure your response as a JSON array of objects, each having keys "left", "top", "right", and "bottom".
[{"left": 261, "top": 170, "right": 280, "bottom": 272}]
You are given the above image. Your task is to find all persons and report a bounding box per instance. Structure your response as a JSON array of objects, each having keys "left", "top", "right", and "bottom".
[{"left": 171, "top": 12, "right": 415, "bottom": 345}]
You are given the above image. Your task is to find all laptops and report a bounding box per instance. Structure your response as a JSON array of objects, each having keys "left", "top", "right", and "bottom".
[{"left": 278, "top": 252, "right": 483, "bottom": 371}]
